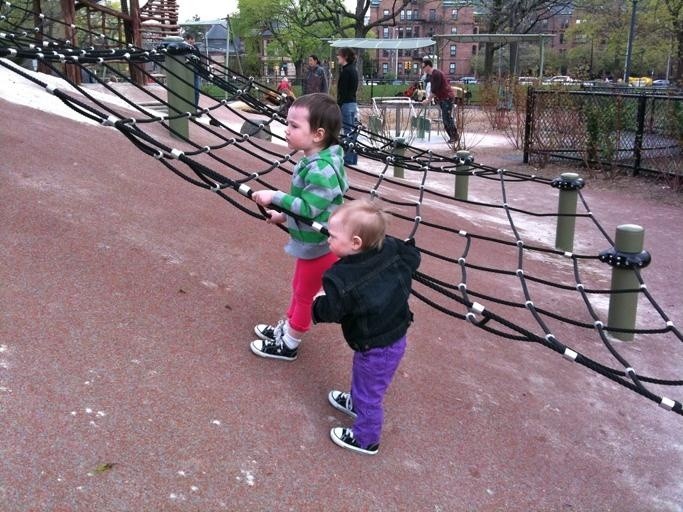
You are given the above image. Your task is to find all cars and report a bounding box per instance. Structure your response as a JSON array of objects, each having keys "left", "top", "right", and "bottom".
[{"left": 518, "top": 76, "right": 581, "bottom": 83}]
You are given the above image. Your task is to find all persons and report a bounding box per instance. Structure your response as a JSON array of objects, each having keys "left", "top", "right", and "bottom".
[
  {"left": 277, "top": 76, "right": 296, "bottom": 101},
  {"left": 308, "top": 197, "right": 421, "bottom": 455},
  {"left": 335, "top": 47, "right": 358, "bottom": 165},
  {"left": 249, "top": 91, "right": 351, "bottom": 361},
  {"left": 184, "top": 33, "right": 201, "bottom": 111},
  {"left": 302, "top": 55, "right": 328, "bottom": 94},
  {"left": 421, "top": 60, "right": 460, "bottom": 143}
]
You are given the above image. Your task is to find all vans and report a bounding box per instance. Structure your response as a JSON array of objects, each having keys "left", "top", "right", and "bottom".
[
  {"left": 653, "top": 80, "right": 670, "bottom": 85},
  {"left": 458, "top": 77, "right": 477, "bottom": 85}
]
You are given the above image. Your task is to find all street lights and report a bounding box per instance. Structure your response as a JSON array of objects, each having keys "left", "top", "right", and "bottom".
[
  {"left": 198, "top": 30, "right": 209, "bottom": 72},
  {"left": 576, "top": 16, "right": 593, "bottom": 72}
]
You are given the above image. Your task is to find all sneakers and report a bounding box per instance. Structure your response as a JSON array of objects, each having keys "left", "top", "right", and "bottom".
[
  {"left": 330, "top": 427, "right": 380, "bottom": 456},
  {"left": 254, "top": 324, "right": 284, "bottom": 340},
  {"left": 448, "top": 134, "right": 460, "bottom": 143},
  {"left": 250, "top": 337, "right": 298, "bottom": 362},
  {"left": 329, "top": 390, "right": 357, "bottom": 418}
]
